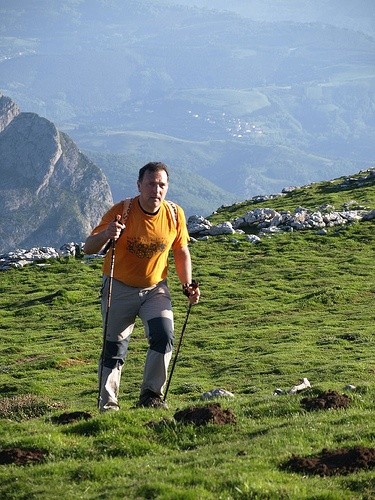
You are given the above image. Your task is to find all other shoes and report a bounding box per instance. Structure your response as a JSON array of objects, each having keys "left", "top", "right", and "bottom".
[{"left": 136, "top": 389, "right": 169, "bottom": 410}]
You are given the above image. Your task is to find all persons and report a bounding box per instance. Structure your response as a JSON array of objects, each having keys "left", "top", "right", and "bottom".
[{"left": 84, "top": 162, "right": 200, "bottom": 411}]
